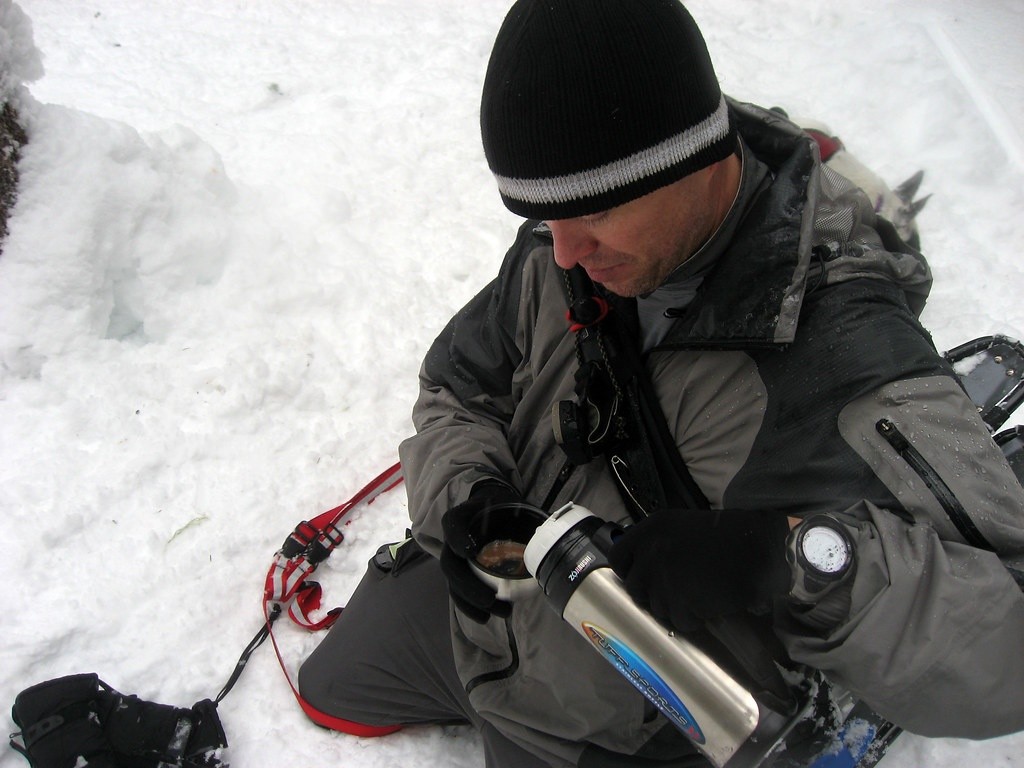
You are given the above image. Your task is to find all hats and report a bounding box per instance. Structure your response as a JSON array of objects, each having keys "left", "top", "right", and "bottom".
[{"left": 480, "top": 0, "right": 736, "bottom": 221}]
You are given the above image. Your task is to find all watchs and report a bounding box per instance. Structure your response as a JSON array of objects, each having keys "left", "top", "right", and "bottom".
[{"left": 782, "top": 512, "right": 856, "bottom": 614}]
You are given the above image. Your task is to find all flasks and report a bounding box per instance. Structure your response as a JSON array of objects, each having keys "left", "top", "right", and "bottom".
[{"left": 521, "top": 500, "right": 817, "bottom": 768}]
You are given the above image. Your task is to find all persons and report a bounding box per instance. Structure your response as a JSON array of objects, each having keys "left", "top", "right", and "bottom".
[{"left": 297, "top": 0, "right": 1024, "bottom": 768}]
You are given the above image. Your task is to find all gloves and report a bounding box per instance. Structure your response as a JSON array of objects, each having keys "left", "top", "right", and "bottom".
[
  {"left": 439, "top": 486, "right": 551, "bottom": 626},
  {"left": 607, "top": 515, "right": 792, "bottom": 634}
]
